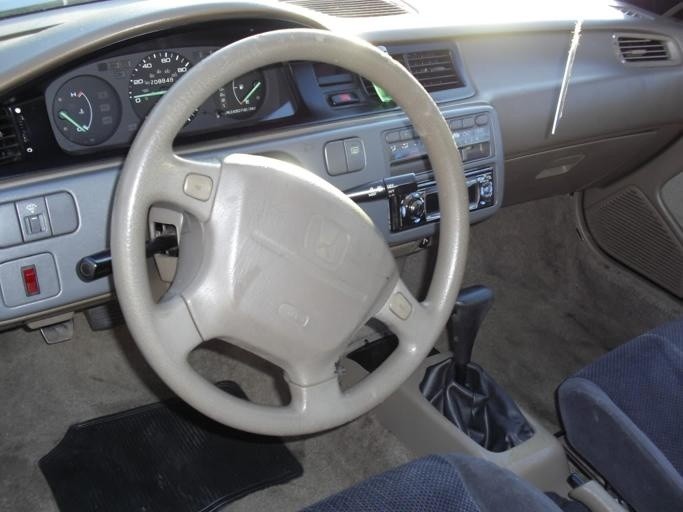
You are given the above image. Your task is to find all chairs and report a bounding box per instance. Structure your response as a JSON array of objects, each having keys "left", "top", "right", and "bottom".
[
  {"left": 554, "top": 316, "right": 683, "bottom": 507},
  {"left": 289, "top": 449, "right": 628, "bottom": 512}
]
